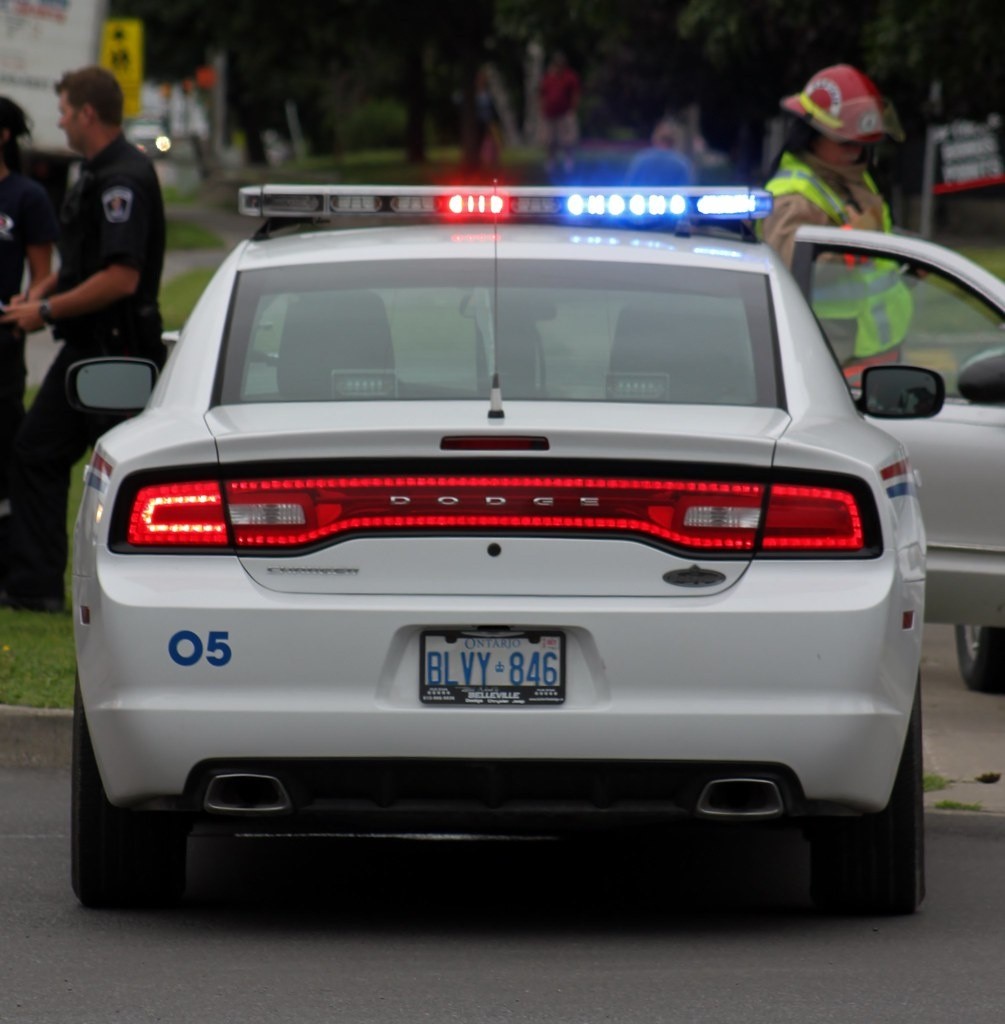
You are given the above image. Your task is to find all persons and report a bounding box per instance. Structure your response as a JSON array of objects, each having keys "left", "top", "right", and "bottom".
[
  {"left": 536, "top": 42, "right": 580, "bottom": 168},
  {"left": 748, "top": 61, "right": 922, "bottom": 402},
  {"left": 0, "top": 65, "right": 168, "bottom": 613},
  {"left": 652, "top": 87, "right": 730, "bottom": 185}
]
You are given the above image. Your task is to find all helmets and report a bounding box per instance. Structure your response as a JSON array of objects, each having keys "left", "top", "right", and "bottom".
[{"left": 781, "top": 65, "right": 885, "bottom": 141}]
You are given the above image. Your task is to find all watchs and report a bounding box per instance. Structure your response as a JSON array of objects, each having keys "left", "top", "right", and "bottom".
[{"left": 39, "top": 295, "right": 58, "bottom": 325}]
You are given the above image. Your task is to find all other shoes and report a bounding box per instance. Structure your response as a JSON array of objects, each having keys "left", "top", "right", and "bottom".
[{"left": 0, "top": 577, "right": 64, "bottom": 610}]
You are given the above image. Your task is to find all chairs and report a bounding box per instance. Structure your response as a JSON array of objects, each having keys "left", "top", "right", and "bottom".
[
  {"left": 280, "top": 288, "right": 415, "bottom": 402},
  {"left": 603, "top": 305, "right": 755, "bottom": 408}
]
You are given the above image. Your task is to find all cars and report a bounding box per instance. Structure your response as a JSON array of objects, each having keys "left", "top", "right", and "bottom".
[
  {"left": 63, "top": 172, "right": 940, "bottom": 917},
  {"left": 784, "top": 224, "right": 1005, "bottom": 695},
  {"left": 124, "top": 121, "right": 168, "bottom": 163}
]
